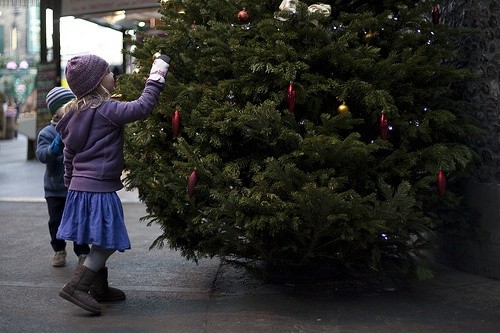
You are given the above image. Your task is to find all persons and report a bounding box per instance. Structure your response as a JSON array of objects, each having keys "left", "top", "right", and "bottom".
[
  {"left": 35, "top": 86, "right": 91, "bottom": 269},
  {"left": 57, "top": 52, "right": 172, "bottom": 314},
  {"left": 0, "top": 94, "right": 19, "bottom": 141}
]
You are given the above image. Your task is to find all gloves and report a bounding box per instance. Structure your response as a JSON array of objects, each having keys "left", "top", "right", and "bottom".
[
  {"left": 147, "top": 55, "right": 171, "bottom": 83},
  {"left": 50, "top": 132, "right": 65, "bottom": 154}
]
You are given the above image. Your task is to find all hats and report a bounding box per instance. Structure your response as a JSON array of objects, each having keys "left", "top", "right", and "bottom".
[
  {"left": 65, "top": 54, "right": 110, "bottom": 99},
  {"left": 46, "top": 87, "right": 76, "bottom": 114}
]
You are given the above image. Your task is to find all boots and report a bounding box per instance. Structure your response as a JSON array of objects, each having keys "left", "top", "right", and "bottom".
[
  {"left": 90, "top": 266, "right": 126, "bottom": 303},
  {"left": 59, "top": 265, "right": 102, "bottom": 313}
]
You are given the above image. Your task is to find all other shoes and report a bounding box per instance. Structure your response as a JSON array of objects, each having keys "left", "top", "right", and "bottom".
[
  {"left": 78, "top": 254, "right": 88, "bottom": 265},
  {"left": 52, "top": 251, "right": 67, "bottom": 267}
]
